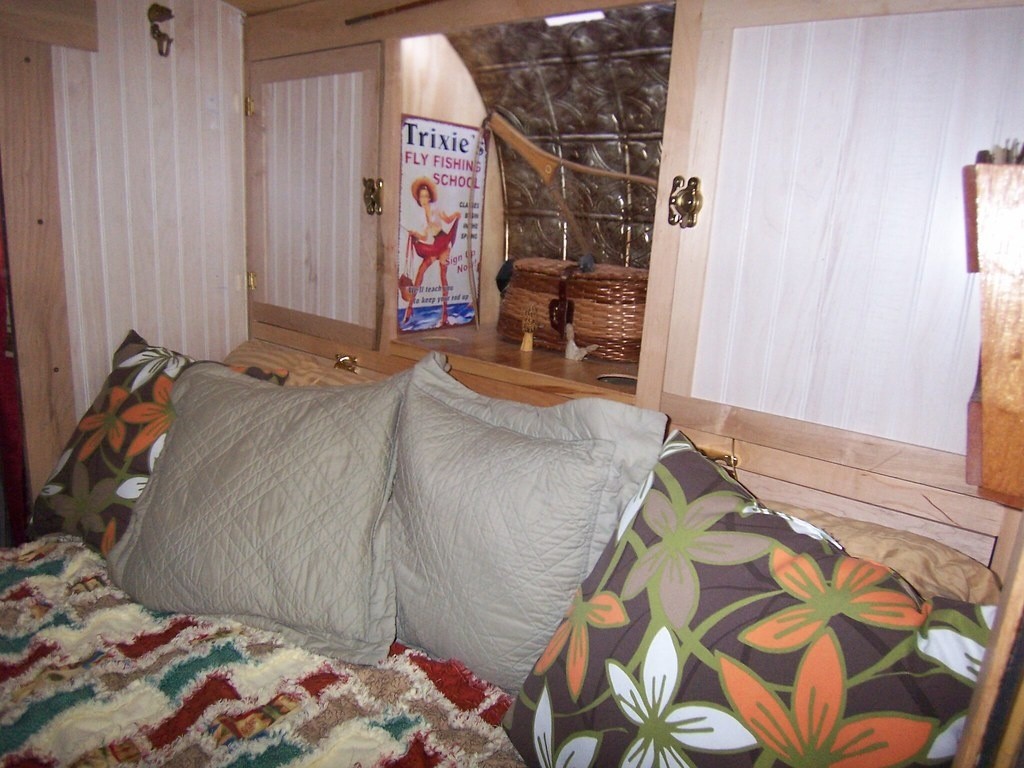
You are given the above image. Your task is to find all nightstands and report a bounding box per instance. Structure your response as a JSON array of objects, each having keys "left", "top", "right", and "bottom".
[
  {"left": 238, "top": 34, "right": 395, "bottom": 379},
  {"left": 634, "top": 1, "right": 1023, "bottom": 495}
]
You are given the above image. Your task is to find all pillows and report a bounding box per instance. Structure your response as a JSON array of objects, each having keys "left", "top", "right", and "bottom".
[
  {"left": 109, "top": 366, "right": 417, "bottom": 664},
  {"left": 501, "top": 427, "right": 1004, "bottom": 768},
  {"left": 389, "top": 348, "right": 671, "bottom": 698},
  {"left": 34, "top": 328, "right": 197, "bottom": 563}
]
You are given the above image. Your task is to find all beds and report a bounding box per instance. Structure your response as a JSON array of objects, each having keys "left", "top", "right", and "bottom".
[{"left": 3, "top": 301, "right": 1024, "bottom": 768}]
